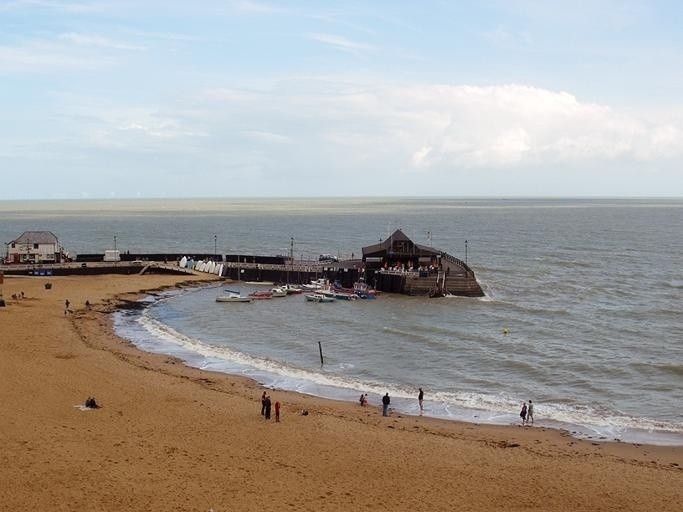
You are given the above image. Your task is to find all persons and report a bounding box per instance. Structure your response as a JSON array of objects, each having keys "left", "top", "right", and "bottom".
[
  {"left": 520, "top": 403, "right": 527, "bottom": 426},
  {"left": 264, "top": 395, "right": 271, "bottom": 420},
  {"left": 419, "top": 388, "right": 424, "bottom": 410},
  {"left": 360, "top": 394, "right": 368, "bottom": 406},
  {"left": 275, "top": 401, "right": 280, "bottom": 422},
  {"left": 526, "top": 400, "right": 534, "bottom": 423},
  {"left": 261, "top": 391, "right": 266, "bottom": 415},
  {"left": 85, "top": 397, "right": 101, "bottom": 408},
  {"left": 382, "top": 393, "right": 390, "bottom": 416}
]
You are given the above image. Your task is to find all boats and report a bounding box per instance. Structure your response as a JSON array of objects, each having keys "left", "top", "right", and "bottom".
[
  {"left": 177, "top": 255, "right": 224, "bottom": 275},
  {"left": 217, "top": 278, "right": 384, "bottom": 303}
]
